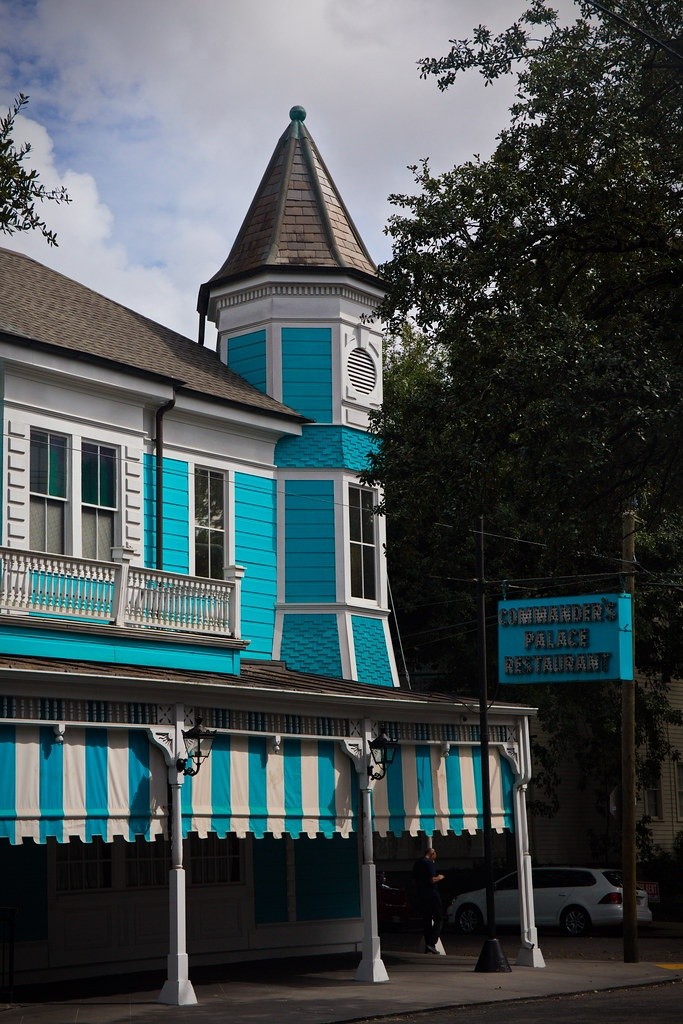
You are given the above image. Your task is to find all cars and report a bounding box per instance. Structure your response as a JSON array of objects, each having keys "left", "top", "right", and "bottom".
[
  {"left": 447, "top": 864, "right": 653, "bottom": 941},
  {"left": 376, "top": 872, "right": 405, "bottom": 928}
]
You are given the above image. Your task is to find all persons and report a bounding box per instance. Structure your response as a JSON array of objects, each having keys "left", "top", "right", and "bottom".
[{"left": 411, "top": 847, "right": 446, "bottom": 953}]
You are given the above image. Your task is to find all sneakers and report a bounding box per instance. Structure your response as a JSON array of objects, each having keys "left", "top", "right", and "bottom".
[{"left": 424, "top": 944, "right": 440, "bottom": 954}]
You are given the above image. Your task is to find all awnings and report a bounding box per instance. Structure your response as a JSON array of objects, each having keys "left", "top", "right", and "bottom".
[{"left": 1, "top": 697, "right": 520, "bottom": 846}]
